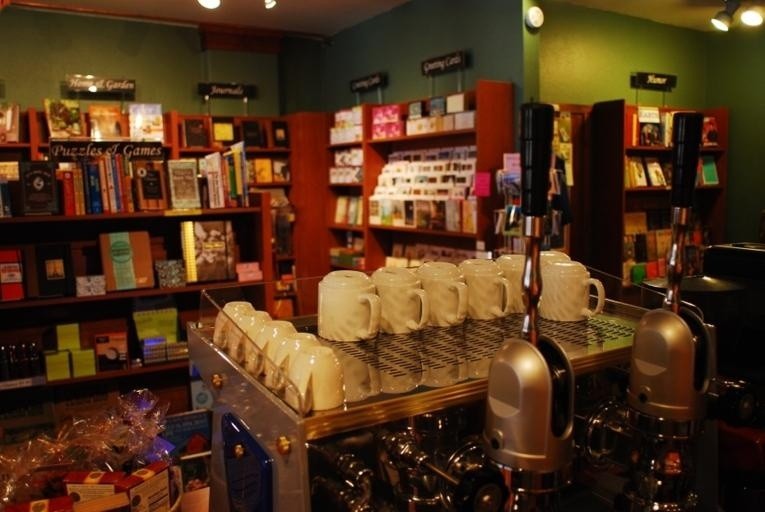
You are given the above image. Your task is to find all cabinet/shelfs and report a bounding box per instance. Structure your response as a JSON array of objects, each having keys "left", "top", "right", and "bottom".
[
  {"left": 1, "top": 113, "right": 273, "bottom": 429},
  {"left": 173, "top": 113, "right": 299, "bottom": 311},
  {"left": 364, "top": 77, "right": 514, "bottom": 273},
  {"left": 327, "top": 105, "right": 366, "bottom": 271},
  {"left": 577, "top": 100, "right": 729, "bottom": 289}
]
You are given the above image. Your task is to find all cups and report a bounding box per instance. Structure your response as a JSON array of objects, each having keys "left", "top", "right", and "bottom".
[
  {"left": 286, "top": 347, "right": 346, "bottom": 417},
  {"left": 539, "top": 259, "right": 606, "bottom": 322},
  {"left": 318, "top": 271, "right": 381, "bottom": 342},
  {"left": 461, "top": 260, "right": 513, "bottom": 321},
  {"left": 424, "top": 328, "right": 469, "bottom": 386},
  {"left": 464, "top": 320, "right": 505, "bottom": 380},
  {"left": 266, "top": 333, "right": 320, "bottom": 391},
  {"left": 504, "top": 313, "right": 528, "bottom": 341},
  {"left": 213, "top": 302, "right": 256, "bottom": 350},
  {"left": 416, "top": 262, "right": 467, "bottom": 328},
  {"left": 378, "top": 334, "right": 429, "bottom": 393},
  {"left": 542, "top": 319, "right": 604, "bottom": 356},
  {"left": 497, "top": 254, "right": 528, "bottom": 314},
  {"left": 538, "top": 250, "right": 570, "bottom": 290},
  {"left": 247, "top": 321, "right": 297, "bottom": 378},
  {"left": 375, "top": 267, "right": 430, "bottom": 335},
  {"left": 333, "top": 343, "right": 381, "bottom": 404},
  {"left": 231, "top": 309, "right": 273, "bottom": 364}
]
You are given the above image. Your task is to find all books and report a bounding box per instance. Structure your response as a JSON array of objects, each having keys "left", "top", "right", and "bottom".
[
  {"left": 98, "top": 229, "right": 155, "bottom": 293},
  {"left": 131, "top": 308, "right": 191, "bottom": 365},
  {"left": 55, "top": 152, "right": 169, "bottom": 216},
  {"left": 631, "top": 106, "right": 674, "bottom": 147},
  {"left": 390, "top": 242, "right": 493, "bottom": 263},
  {"left": 211, "top": 114, "right": 235, "bottom": 143},
  {"left": 127, "top": 100, "right": 165, "bottom": 143},
  {"left": 0, "top": 98, "right": 21, "bottom": 146},
  {"left": 181, "top": 218, "right": 264, "bottom": 285},
  {"left": 624, "top": 153, "right": 672, "bottom": 190},
  {"left": 89, "top": 104, "right": 130, "bottom": 143},
  {"left": 240, "top": 117, "right": 269, "bottom": 148},
  {"left": 178, "top": 115, "right": 211, "bottom": 150},
  {"left": 22, "top": 238, "right": 76, "bottom": 301},
  {"left": 3, "top": 157, "right": 58, "bottom": 220},
  {"left": 701, "top": 115, "right": 720, "bottom": 149},
  {"left": 41, "top": 97, "right": 88, "bottom": 139},
  {"left": 42, "top": 311, "right": 132, "bottom": 384},
  {"left": 0, "top": 247, "right": 25, "bottom": 304},
  {"left": 334, "top": 193, "right": 363, "bottom": 226},
  {"left": 405, "top": 92, "right": 476, "bottom": 140},
  {"left": 329, "top": 107, "right": 362, "bottom": 145},
  {"left": 271, "top": 119, "right": 293, "bottom": 150},
  {"left": 368, "top": 146, "right": 476, "bottom": 235},
  {"left": 205, "top": 142, "right": 245, "bottom": 210},
  {"left": 696, "top": 156, "right": 719, "bottom": 187},
  {"left": 244, "top": 156, "right": 292, "bottom": 187},
  {"left": 329, "top": 147, "right": 365, "bottom": 184},
  {"left": 168, "top": 159, "right": 202, "bottom": 212},
  {"left": 369, "top": 93, "right": 407, "bottom": 141},
  {"left": 327, "top": 234, "right": 365, "bottom": 270},
  {"left": 622, "top": 210, "right": 673, "bottom": 287},
  {"left": 676, "top": 208, "right": 713, "bottom": 278}
]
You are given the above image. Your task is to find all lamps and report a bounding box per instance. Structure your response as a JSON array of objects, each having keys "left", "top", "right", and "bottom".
[
  {"left": 709, "top": 3, "right": 740, "bottom": 32},
  {"left": 743, "top": 2, "right": 765, "bottom": 27}
]
